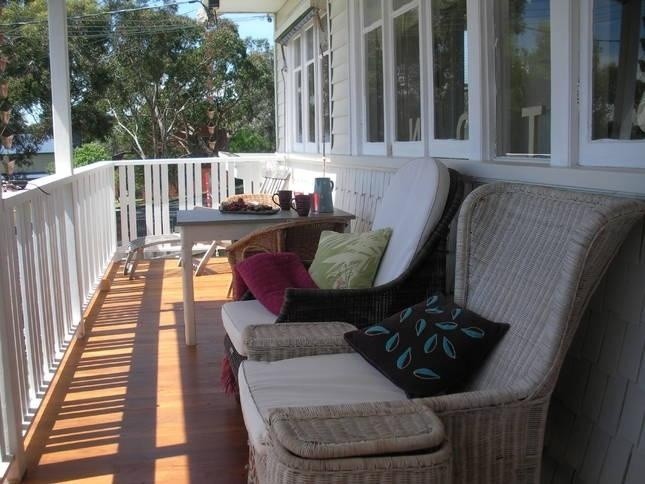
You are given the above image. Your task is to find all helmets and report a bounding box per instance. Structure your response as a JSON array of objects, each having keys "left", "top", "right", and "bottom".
[{"left": 223, "top": 205, "right": 280, "bottom": 215}]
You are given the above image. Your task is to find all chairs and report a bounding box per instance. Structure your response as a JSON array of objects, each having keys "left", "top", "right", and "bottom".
[
  {"left": 238, "top": 180, "right": 645, "bottom": 484},
  {"left": 123, "top": 167, "right": 293, "bottom": 279},
  {"left": 222, "top": 157, "right": 469, "bottom": 405}
]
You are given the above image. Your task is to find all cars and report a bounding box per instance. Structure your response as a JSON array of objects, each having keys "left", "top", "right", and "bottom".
[{"left": 272, "top": 177, "right": 334, "bottom": 217}]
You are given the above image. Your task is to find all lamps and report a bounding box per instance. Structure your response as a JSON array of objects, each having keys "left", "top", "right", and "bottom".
[{"left": 123, "top": 167, "right": 293, "bottom": 279}]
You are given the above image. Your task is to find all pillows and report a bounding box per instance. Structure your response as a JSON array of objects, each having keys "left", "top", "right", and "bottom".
[
  {"left": 309, "top": 226, "right": 392, "bottom": 292},
  {"left": 343, "top": 292, "right": 511, "bottom": 400},
  {"left": 236, "top": 252, "right": 322, "bottom": 316}
]
